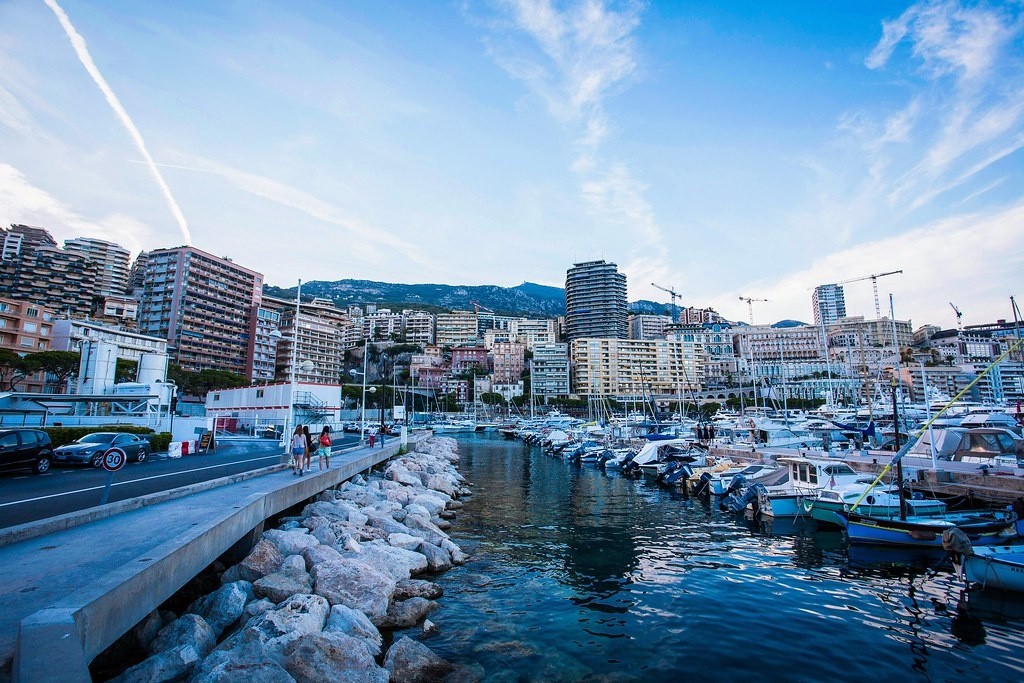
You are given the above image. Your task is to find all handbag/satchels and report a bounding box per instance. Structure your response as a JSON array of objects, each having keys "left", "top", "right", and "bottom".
[
  {"left": 287, "top": 453, "right": 297, "bottom": 468},
  {"left": 309, "top": 441, "right": 317, "bottom": 453},
  {"left": 320, "top": 432, "right": 332, "bottom": 446}
]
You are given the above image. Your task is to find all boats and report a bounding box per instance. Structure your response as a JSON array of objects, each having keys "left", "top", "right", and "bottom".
[{"left": 425, "top": 292, "right": 1024, "bottom": 602}]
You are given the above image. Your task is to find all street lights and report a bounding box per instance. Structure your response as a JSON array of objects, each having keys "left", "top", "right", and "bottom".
[
  {"left": 348, "top": 337, "right": 377, "bottom": 443},
  {"left": 269, "top": 279, "right": 315, "bottom": 455}
]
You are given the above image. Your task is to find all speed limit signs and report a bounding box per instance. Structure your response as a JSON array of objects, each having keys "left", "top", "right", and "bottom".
[{"left": 101, "top": 447, "right": 127, "bottom": 472}]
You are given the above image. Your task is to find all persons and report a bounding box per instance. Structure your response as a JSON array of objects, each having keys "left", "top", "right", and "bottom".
[
  {"left": 289, "top": 424, "right": 302, "bottom": 470},
  {"left": 379, "top": 424, "right": 386, "bottom": 447},
  {"left": 240, "top": 423, "right": 246, "bottom": 435},
  {"left": 318, "top": 425, "right": 331, "bottom": 471},
  {"left": 707, "top": 424, "right": 715, "bottom": 446},
  {"left": 696, "top": 424, "right": 704, "bottom": 444},
  {"left": 244, "top": 423, "right": 250, "bottom": 435},
  {"left": 703, "top": 422, "right": 710, "bottom": 446},
  {"left": 367, "top": 423, "right": 377, "bottom": 447},
  {"left": 289, "top": 425, "right": 308, "bottom": 476},
  {"left": 302, "top": 426, "right": 316, "bottom": 471}
]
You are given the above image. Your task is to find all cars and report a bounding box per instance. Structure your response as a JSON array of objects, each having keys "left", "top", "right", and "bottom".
[
  {"left": 341, "top": 411, "right": 430, "bottom": 438},
  {"left": 53, "top": 432, "right": 151, "bottom": 469}
]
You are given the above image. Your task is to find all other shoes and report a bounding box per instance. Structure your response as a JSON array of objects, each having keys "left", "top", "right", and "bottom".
[
  {"left": 292, "top": 471, "right": 297, "bottom": 475},
  {"left": 299, "top": 469, "right": 304, "bottom": 476},
  {"left": 306, "top": 469, "right": 312, "bottom": 472},
  {"left": 326, "top": 462, "right": 330, "bottom": 469}
]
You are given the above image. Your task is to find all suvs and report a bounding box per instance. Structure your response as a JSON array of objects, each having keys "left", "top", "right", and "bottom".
[{"left": 0, "top": 429, "right": 54, "bottom": 476}]
